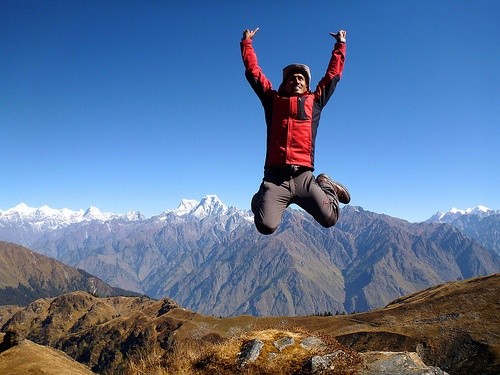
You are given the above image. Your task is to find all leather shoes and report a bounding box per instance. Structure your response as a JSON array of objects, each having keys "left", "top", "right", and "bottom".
[
  {"left": 316, "top": 173, "right": 350, "bottom": 204},
  {"left": 251, "top": 181, "right": 264, "bottom": 214}
]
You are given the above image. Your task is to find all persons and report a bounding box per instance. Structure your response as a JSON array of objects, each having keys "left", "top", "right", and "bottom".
[{"left": 239, "top": 28, "right": 351, "bottom": 235}]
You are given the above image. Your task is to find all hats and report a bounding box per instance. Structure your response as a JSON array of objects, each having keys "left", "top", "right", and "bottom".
[{"left": 283, "top": 64, "right": 310, "bottom": 90}]
